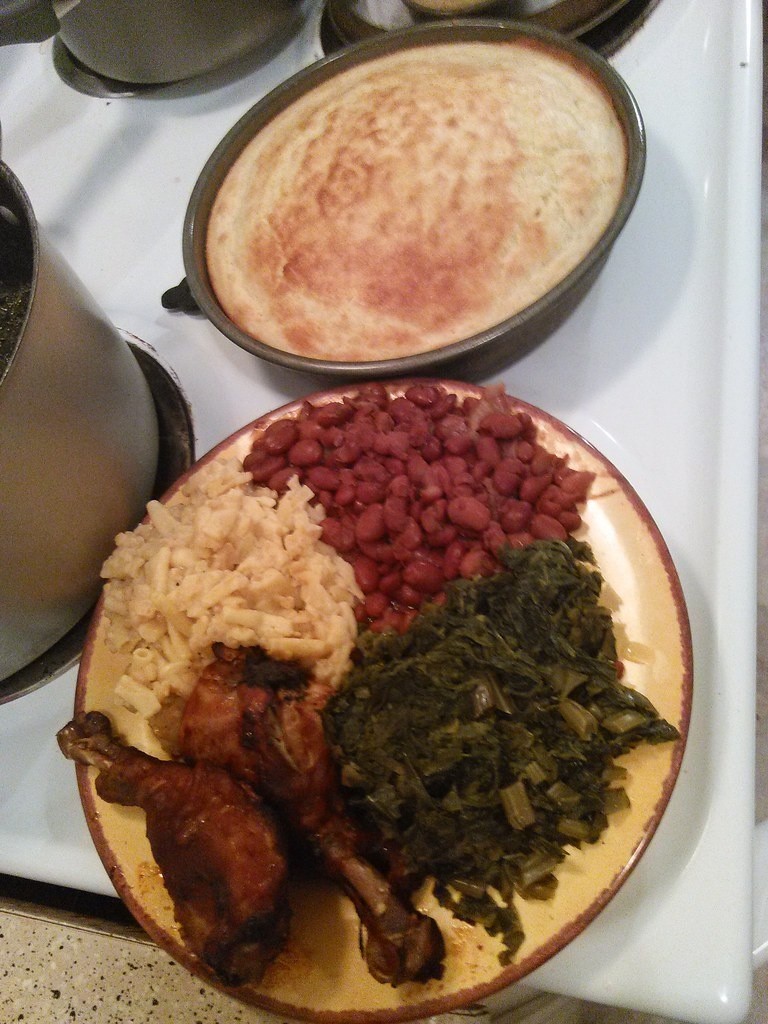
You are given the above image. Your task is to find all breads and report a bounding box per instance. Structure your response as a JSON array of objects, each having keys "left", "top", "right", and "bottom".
[{"left": 205, "top": 38, "right": 632, "bottom": 363}]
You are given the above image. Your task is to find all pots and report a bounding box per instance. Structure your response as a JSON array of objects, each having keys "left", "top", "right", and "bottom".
[
  {"left": 0, "top": 0, "right": 313, "bottom": 85},
  {"left": 0, "top": 118, "right": 159, "bottom": 683}
]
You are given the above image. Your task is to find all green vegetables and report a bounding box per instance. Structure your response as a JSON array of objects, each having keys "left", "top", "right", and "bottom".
[{"left": 320, "top": 534, "right": 681, "bottom": 964}]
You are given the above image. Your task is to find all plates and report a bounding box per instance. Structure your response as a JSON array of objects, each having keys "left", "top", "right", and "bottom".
[
  {"left": 317, "top": 0, "right": 663, "bottom": 63},
  {"left": 1, "top": 326, "right": 197, "bottom": 704},
  {"left": 75, "top": 377, "right": 693, "bottom": 1024},
  {"left": 52, "top": 0, "right": 324, "bottom": 100},
  {"left": 180, "top": 19, "right": 645, "bottom": 381}
]
